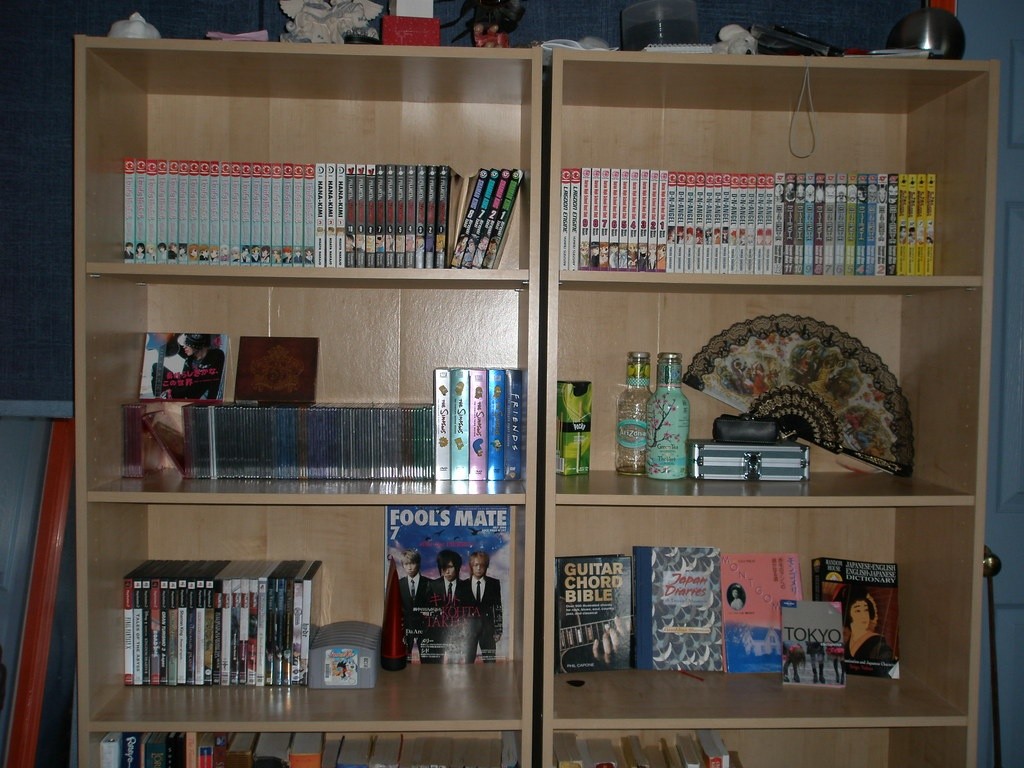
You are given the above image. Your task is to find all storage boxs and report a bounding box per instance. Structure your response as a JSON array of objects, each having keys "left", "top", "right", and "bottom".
[
  {"left": 382, "top": 15, "right": 440, "bottom": 46},
  {"left": 308, "top": 620, "right": 383, "bottom": 690},
  {"left": 234, "top": 336, "right": 320, "bottom": 407},
  {"left": 689, "top": 439, "right": 811, "bottom": 483},
  {"left": 388, "top": 0, "right": 434, "bottom": 18}
]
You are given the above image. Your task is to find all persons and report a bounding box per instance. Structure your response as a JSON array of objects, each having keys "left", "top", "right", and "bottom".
[
  {"left": 396, "top": 548, "right": 503, "bottom": 663},
  {"left": 151, "top": 334, "right": 225, "bottom": 399}
]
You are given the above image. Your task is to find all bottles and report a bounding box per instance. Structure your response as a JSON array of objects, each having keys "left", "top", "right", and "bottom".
[
  {"left": 644, "top": 351, "right": 691, "bottom": 480},
  {"left": 616, "top": 350, "right": 653, "bottom": 476}
]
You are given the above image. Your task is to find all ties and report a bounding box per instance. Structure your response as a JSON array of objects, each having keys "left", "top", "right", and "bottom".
[
  {"left": 411, "top": 580, "right": 415, "bottom": 597},
  {"left": 476, "top": 581, "right": 481, "bottom": 600},
  {"left": 448, "top": 583, "right": 453, "bottom": 601}
]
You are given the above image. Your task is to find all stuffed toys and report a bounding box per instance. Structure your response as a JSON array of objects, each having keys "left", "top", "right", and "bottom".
[{"left": 712, "top": 23, "right": 758, "bottom": 55}]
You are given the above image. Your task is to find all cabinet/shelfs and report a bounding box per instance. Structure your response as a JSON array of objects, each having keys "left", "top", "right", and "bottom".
[
  {"left": 74, "top": 34, "right": 541, "bottom": 768},
  {"left": 541, "top": 46, "right": 1001, "bottom": 768}
]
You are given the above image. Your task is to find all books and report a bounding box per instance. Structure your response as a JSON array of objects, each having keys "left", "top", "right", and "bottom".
[
  {"left": 552, "top": 730, "right": 744, "bottom": 768},
  {"left": 560, "top": 167, "right": 936, "bottom": 277},
  {"left": 644, "top": 42, "right": 715, "bottom": 54},
  {"left": 555, "top": 546, "right": 901, "bottom": 687},
  {"left": 100, "top": 732, "right": 520, "bottom": 768},
  {"left": 385, "top": 504, "right": 511, "bottom": 665},
  {"left": 123, "top": 558, "right": 324, "bottom": 687},
  {"left": 123, "top": 157, "right": 524, "bottom": 270},
  {"left": 123, "top": 330, "right": 524, "bottom": 482}
]
